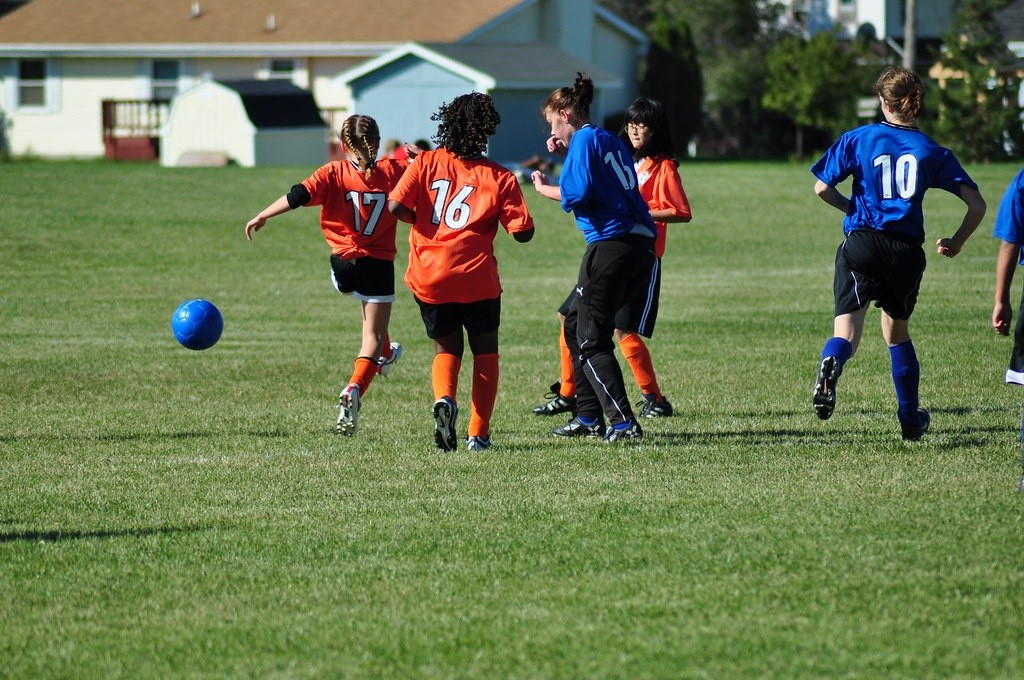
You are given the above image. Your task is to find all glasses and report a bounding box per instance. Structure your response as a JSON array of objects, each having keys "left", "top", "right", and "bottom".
[{"left": 624, "top": 123, "right": 650, "bottom": 135}]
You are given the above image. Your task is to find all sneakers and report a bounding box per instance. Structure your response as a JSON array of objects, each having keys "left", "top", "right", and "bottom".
[
  {"left": 551, "top": 413, "right": 606, "bottom": 437},
  {"left": 429, "top": 396, "right": 459, "bottom": 457},
  {"left": 898, "top": 407, "right": 929, "bottom": 442},
  {"left": 636, "top": 393, "right": 673, "bottom": 418},
  {"left": 603, "top": 419, "right": 643, "bottom": 441},
  {"left": 531, "top": 382, "right": 577, "bottom": 415},
  {"left": 336, "top": 383, "right": 362, "bottom": 438},
  {"left": 377, "top": 341, "right": 402, "bottom": 377},
  {"left": 466, "top": 432, "right": 491, "bottom": 451},
  {"left": 812, "top": 356, "right": 842, "bottom": 422}
]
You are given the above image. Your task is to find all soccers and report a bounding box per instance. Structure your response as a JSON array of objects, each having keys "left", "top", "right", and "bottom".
[{"left": 171, "top": 299, "right": 224, "bottom": 351}]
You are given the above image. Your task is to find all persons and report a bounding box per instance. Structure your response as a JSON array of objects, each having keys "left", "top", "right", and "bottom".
[
  {"left": 246, "top": 72, "right": 692, "bottom": 436},
  {"left": 391, "top": 90, "right": 534, "bottom": 449},
  {"left": 813, "top": 66, "right": 987, "bottom": 442},
  {"left": 991, "top": 169, "right": 1024, "bottom": 460}
]
